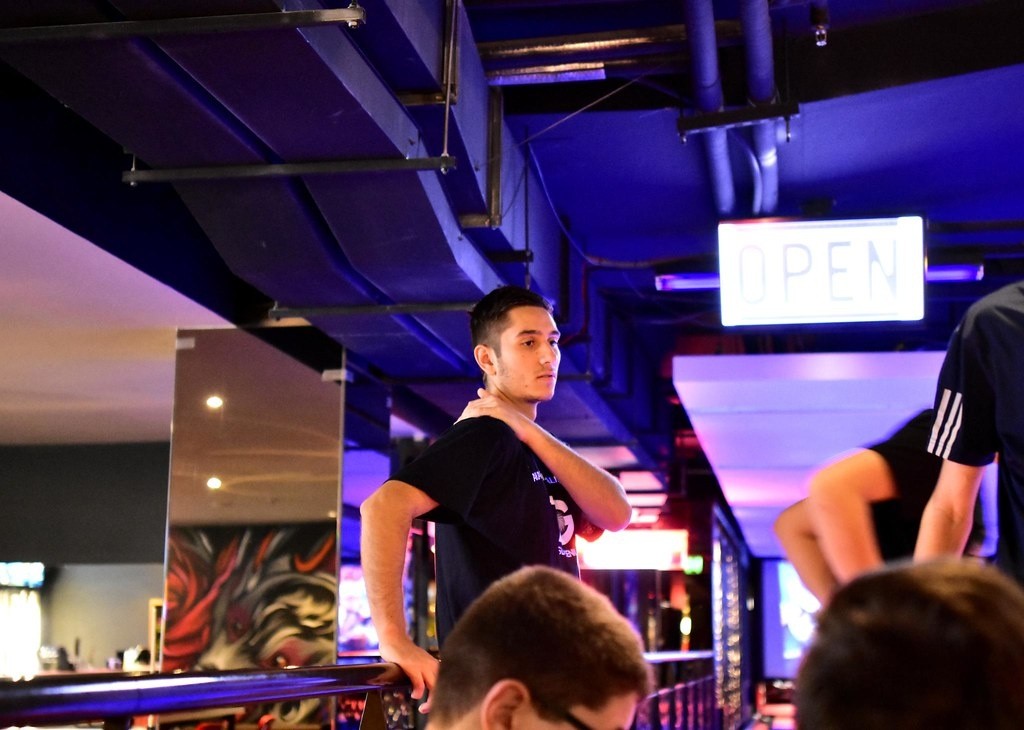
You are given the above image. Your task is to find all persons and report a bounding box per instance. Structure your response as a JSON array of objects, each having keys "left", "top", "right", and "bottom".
[
  {"left": 773, "top": 408, "right": 987, "bottom": 607},
  {"left": 423, "top": 564, "right": 653, "bottom": 730},
  {"left": 794, "top": 562, "right": 1024, "bottom": 729},
  {"left": 916, "top": 279, "right": 1024, "bottom": 590},
  {"left": 358, "top": 286, "right": 633, "bottom": 714}
]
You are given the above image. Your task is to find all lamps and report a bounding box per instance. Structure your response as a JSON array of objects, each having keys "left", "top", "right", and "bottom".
[{"left": 718, "top": 213, "right": 925, "bottom": 327}]
